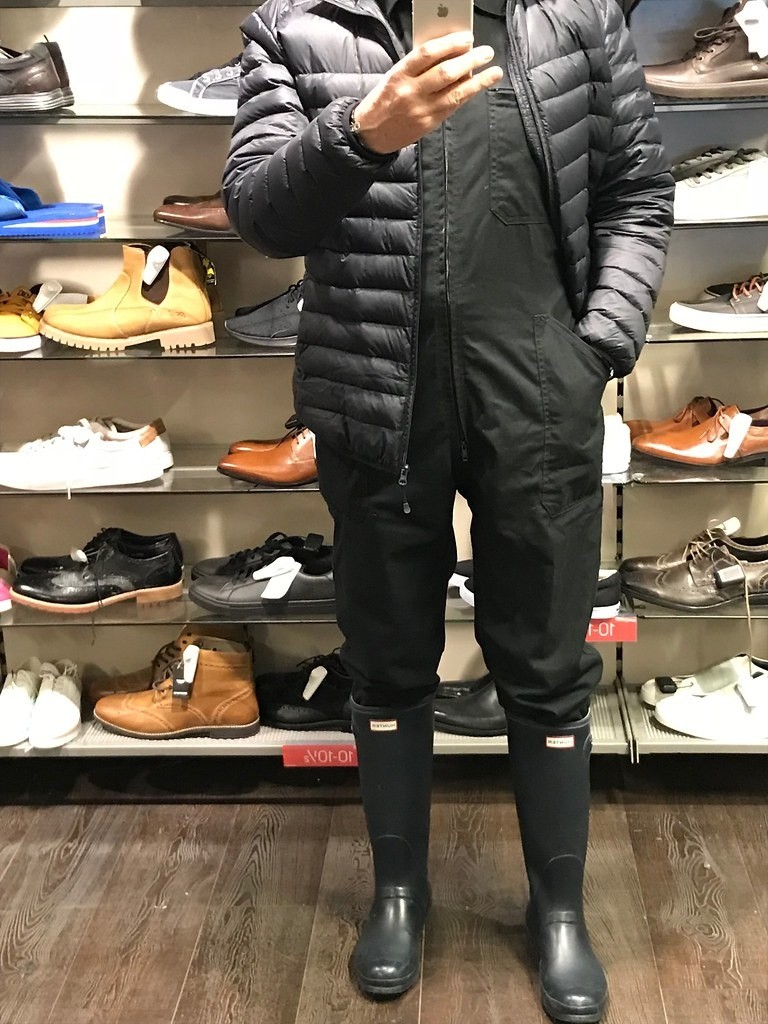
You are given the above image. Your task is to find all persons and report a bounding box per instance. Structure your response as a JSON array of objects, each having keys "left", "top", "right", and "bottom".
[{"left": 221, "top": 0, "right": 676, "bottom": 1022}]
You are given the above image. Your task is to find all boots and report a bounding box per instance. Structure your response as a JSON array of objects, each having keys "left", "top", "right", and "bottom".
[
  {"left": 351, "top": 677, "right": 440, "bottom": 995},
  {"left": 506, "top": 707, "right": 608, "bottom": 1023}
]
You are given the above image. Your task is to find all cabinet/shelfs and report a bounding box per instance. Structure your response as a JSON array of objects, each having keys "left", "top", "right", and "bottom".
[
  {"left": 0, "top": 0, "right": 635, "bottom": 769},
  {"left": 617, "top": 0, "right": 768, "bottom": 763}
]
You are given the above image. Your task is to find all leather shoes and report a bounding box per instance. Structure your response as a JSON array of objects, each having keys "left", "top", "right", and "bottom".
[
  {"left": 625, "top": 393, "right": 768, "bottom": 442},
  {"left": 621, "top": 547, "right": 768, "bottom": 608},
  {"left": 433, "top": 680, "right": 509, "bottom": 735},
  {"left": 39, "top": 245, "right": 215, "bottom": 350},
  {"left": 94, "top": 636, "right": 260, "bottom": 739},
  {"left": 438, "top": 673, "right": 495, "bottom": 697},
  {"left": 619, "top": 528, "right": 768, "bottom": 573},
  {"left": 631, "top": 405, "right": 768, "bottom": 467},
  {"left": 220, "top": 427, "right": 320, "bottom": 488},
  {"left": 9, "top": 542, "right": 184, "bottom": 613},
  {"left": 228, "top": 435, "right": 291, "bottom": 453},
  {"left": 17, "top": 528, "right": 183, "bottom": 575},
  {"left": 154, "top": 197, "right": 231, "bottom": 234}
]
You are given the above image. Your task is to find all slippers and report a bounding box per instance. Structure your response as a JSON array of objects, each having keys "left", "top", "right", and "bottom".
[
  {"left": 0, "top": 179, "right": 107, "bottom": 233},
  {"left": 0, "top": 190, "right": 99, "bottom": 234}
]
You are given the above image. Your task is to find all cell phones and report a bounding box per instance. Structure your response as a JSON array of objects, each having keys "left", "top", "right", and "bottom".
[{"left": 412, "top": 0, "right": 474, "bottom": 81}]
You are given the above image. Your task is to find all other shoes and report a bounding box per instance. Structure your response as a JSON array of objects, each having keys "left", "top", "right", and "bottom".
[
  {"left": 0, "top": 46, "right": 66, "bottom": 111},
  {"left": 602, "top": 424, "right": 628, "bottom": 474},
  {"left": 449, "top": 559, "right": 474, "bottom": 581},
  {"left": 0, "top": 41, "right": 74, "bottom": 106},
  {"left": 258, "top": 654, "right": 353, "bottom": 732},
  {"left": 158, "top": 64, "right": 243, "bottom": 116},
  {"left": 604, "top": 412, "right": 623, "bottom": 423},
  {"left": 90, "top": 625, "right": 253, "bottom": 701},
  {"left": 655, "top": 672, "right": 768, "bottom": 741},
  {"left": 0, "top": 287, "right": 43, "bottom": 353},
  {"left": 29, "top": 659, "right": 82, "bottom": 749},
  {"left": 460, "top": 568, "right": 619, "bottom": 620},
  {"left": 190, "top": 53, "right": 245, "bottom": 79},
  {"left": 163, "top": 192, "right": 222, "bottom": 206},
  {"left": 255, "top": 650, "right": 348, "bottom": 686},
  {"left": 0, "top": 656, "right": 41, "bottom": 747},
  {"left": 641, "top": 653, "right": 768, "bottom": 706}
]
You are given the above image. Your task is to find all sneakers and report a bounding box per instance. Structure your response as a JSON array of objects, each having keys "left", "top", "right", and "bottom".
[
  {"left": 670, "top": 147, "right": 739, "bottom": 178},
  {"left": 225, "top": 286, "right": 305, "bottom": 347},
  {"left": 4, "top": 416, "right": 174, "bottom": 469},
  {"left": 639, "top": 0, "right": 768, "bottom": 100},
  {"left": 673, "top": 147, "right": 768, "bottom": 222},
  {"left": 669, "top": 279, "right": 768, "bottom": 333},
  {"left": 701, "top": 273, "right": 768, "bottom": 300},
  {"left": 189, "top": 551, "right": 338, "bottom": 615},
  {"left": 235, "top": 281, "right": 303, "bottom": 317},
  {"left": 0, "top": 424, "right": 165, "bottom": 500},
  {"left": 191, "top": 535, "right": 339, "bottom": 578}
]
